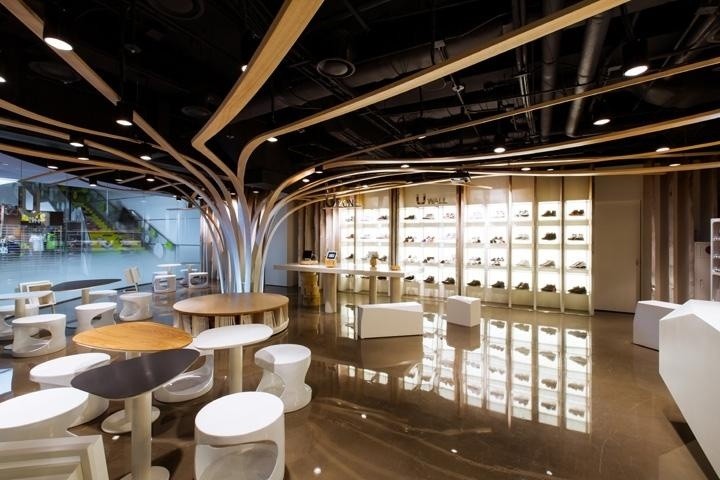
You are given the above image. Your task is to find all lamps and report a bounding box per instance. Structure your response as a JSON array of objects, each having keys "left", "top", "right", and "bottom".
[
  {"left": 139, "top": 143, "right": 152, "bottom": 161},
  {"left": 48, "top": 159, "right": 58, "bottom": 169},
  {"left": 617, "top": 31, "right": 648, "bottom": 78},
  {"left": 492, "top": 134, "right": 506, "bottom": 153},
  {"left": 176, "top": 196, "right": 181, "bottom": 200},
  {"left": 187, "top": 202, "right": 192, "bottom": 209},
  {"left": 42, "top": 9, "right": 78, "bottom": 51},
  {"left": 145, "top": 173, "right": 155, "bottom": 182},
  {"left": 654, "top": 135, "right": 670, "bottom": 153},
  {"left": 113, "top": 100, "right": 132, "bottom": 126},
  {"left": 592, "top": 104, "right": 610, "bottom": 126},
  {"left": 77, "top": 147, "right": 90, "bottom": 160},
  {"left": 315, "top": 165, "right": 324, "bottom": 173},
  {"left": 68, "top": 132, "right": 84, "bottom": 147},
  {"left": 89, "top": 176, "right": 97, "bottom": 187}
]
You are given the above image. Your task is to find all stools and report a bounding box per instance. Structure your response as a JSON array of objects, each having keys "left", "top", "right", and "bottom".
[
  {"left": 180, "top": 269, "right": 199, "bottom": 284},
  {"left": 447, "top": 295, "right": 481, "bottom": 327},
  {"left": 632, "top": 299, "right": 682, "bottom": 351},
  {"left": 120, "top": 292, "right": 154, "bottom": 321},
  {"left": 152, "top": 271, "right": 169, "bottom": 289},
  {"left": 75, "top": 302, "right": 117, "bottom": 333},
  {"left": 188, "top": 272, "right": 209, "bottom": 288},
  {"left": 89, "top": 290, "right": 117, "bottom": 301},
  {"left": 11, "top": 313, "right": 67, "bottom": 358},
  {"left": 358, "top": 300, "right": 423, "bottom": 339},
  {"left": 0, "top": 305, "right": 34, "bottom": 336},
  {"left": 153, "top": 275, "right": 176, "bottom": 294},
  {"left": 29, "top": 353, "right": 111, "bottom": 428},
  {"left": 195, "top": 391, "right": 285, "bottom": 480},
  {"left": 153, "top": 339, "right": 215, "bottom": 403},
  {"left": 0, "top": 387, "right": 90, "bottom": 437}
]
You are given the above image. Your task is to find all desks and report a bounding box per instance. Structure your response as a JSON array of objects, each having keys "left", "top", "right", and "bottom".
[
  {"left": 193, "top": 324, "right": 273, "bottom": 394},
  {"left": 271, "top": 261, "right": 405, "bottom": 313},
  {"left": 172, "top": 293, "right": 290, "bottom": 335},
  {"left": 72, "top": 323, "right": 192, "bottom": 434},
  {"left": 70, "top": 350, "right": 200, "bottom": 480},
  {"left": 0, "top": 291, "right": 52, "bottom": 340},
  {"left": 157, "top": 264, "right": 182, "bottom": 274},
  {"left": 50, "top": 278, "right": 122, "bottom": 303}
]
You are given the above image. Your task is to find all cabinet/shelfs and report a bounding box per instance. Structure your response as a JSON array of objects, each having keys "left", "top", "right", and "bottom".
[
  {"left": 708, "top": 219, "right": 720, "bottom": 302},
  {"left": 340, "top": 200, "right": 591, "bottom": 316}
]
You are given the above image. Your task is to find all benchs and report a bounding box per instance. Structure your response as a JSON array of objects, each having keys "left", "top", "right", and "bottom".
[{"left": 254, "top": 344, "right": 312, "bottom": 413}]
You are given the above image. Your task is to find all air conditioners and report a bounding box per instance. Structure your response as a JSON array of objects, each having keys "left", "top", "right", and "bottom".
[{"left": 694, "top": 242, "right": 711, "bottom": 301}]
